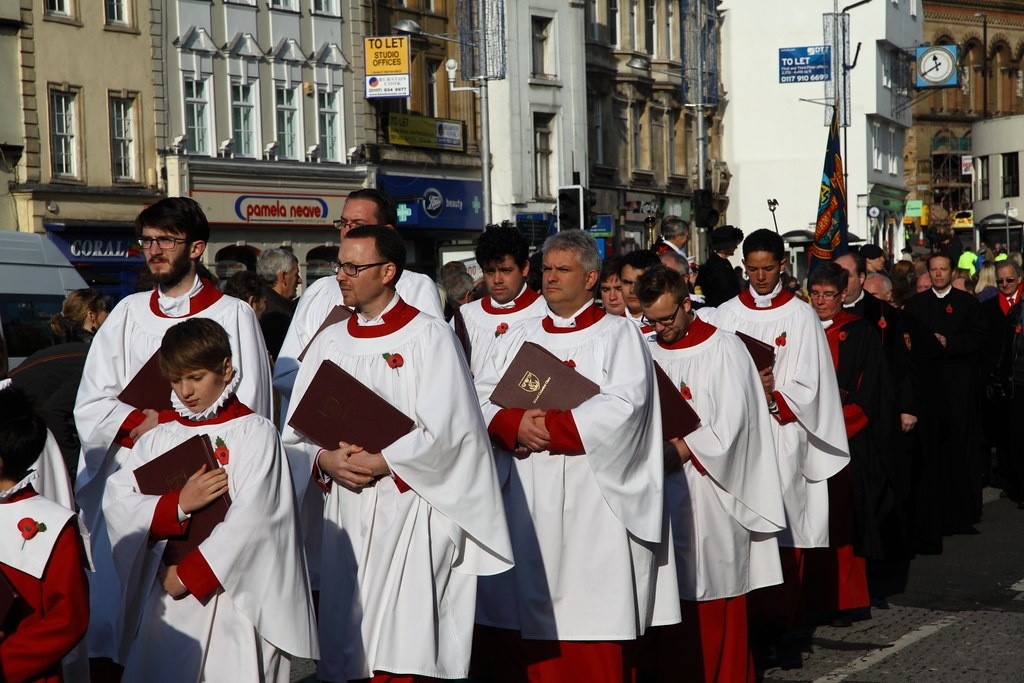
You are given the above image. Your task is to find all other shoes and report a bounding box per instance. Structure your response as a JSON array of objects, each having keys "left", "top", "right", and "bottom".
[
  {"left": 774, "top": 627, "right": 803, "bottom": 670},
  {"left": 832, "top": 618, "right": 852, "bottom": 627},
  {"left": 757, "top": 626, "right": 780, "bottom": 665}
]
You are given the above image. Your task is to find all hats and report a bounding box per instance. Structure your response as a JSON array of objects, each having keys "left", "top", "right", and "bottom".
[
  {"left": 978, "top": 248, "right": 987, "bottom": 254},
  {"left": 711, "top": 225, "right": 743, "bottom": 252},
  {"left": 861, "top": 244, "right": 884, "bottom": 259}
]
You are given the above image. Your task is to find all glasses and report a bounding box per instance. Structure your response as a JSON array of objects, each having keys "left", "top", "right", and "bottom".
[
  {"left": 333, "top": 219, "right": 357, "bottom": 231},
  {"left": 641, "top": 302, "right": 681, "bottom": 326},
  {"left": 996, "top": 278, "right": 1014, "bottom": 284},
  {"left": 329, "top": 258, "right": 388, "bottom": 276},
  {"left": 811, "top": 289, "right": 845, "bottom": 301},
  {"left": 135, "top": 235, "right": 187, "bottom": 249}
]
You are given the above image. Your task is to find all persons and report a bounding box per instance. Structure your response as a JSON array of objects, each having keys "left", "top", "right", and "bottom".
[{"left": 0, "top": 189, "right": 1024, "bottom": 681}]
